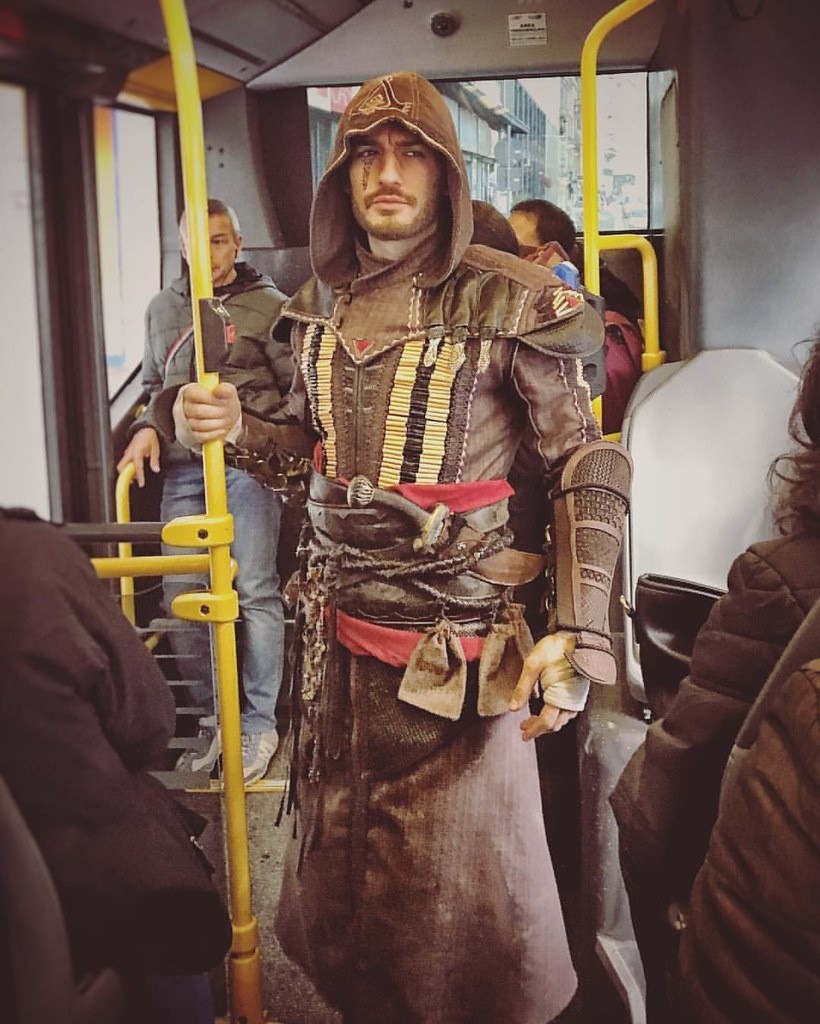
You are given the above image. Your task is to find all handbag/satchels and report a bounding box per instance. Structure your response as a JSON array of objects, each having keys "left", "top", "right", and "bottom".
[{"left": 635, "top": 573, "right": 728, "bottom": 705}]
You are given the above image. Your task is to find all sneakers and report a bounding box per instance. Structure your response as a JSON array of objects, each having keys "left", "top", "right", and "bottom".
[{"left": 175, "top": 716, "right": 279, "bottom": 788}]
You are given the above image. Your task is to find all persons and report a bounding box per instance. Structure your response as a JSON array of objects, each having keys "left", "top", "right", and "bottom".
[
  {"left": 116, "top": 196, "right": 296, "bottom": 786},
  {"left": 467, "top": 198, "right": 642, "bottom": 627},
  {"left": 171, "top": 70, "right": 632, "bottom": 1023},
  {"left": 606, "top": 322, "right": 820, "bottom": 1023},
  {"left": 0, "top": 507, "right": 231, "bottom": 975}
]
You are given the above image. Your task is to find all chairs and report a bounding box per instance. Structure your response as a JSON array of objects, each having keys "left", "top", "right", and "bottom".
[
  {"left": 619, "top": 358, "right": 692, "bottom": 705},
  {"left": 627, "top": 345, "right": 816, "bottom": 666},
  {"left": 0, "top": 774, "right": 140, "bottom": 1024}
]
